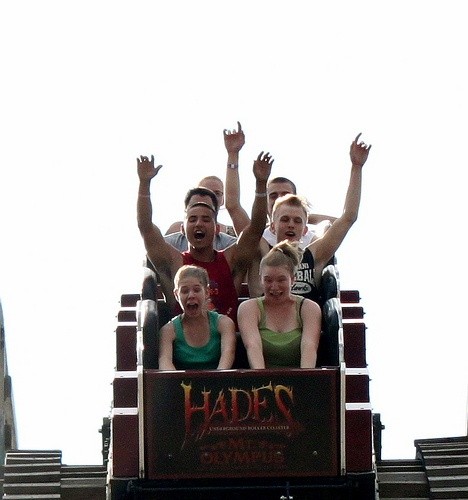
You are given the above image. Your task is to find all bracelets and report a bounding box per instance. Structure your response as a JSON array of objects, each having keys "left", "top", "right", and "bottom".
[
  {"left": 139, "top": 192, "right": 150, "bottom": 199},
  {"left": 255, "top": 192, "right": 268, "bottom": 199}
]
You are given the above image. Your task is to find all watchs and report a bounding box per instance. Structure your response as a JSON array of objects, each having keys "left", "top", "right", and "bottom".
[{"left": 226, "top": 162, "right": 238, "bottom": 170}]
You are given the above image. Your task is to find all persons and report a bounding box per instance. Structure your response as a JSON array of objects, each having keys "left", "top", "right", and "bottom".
[
  {"left": 137, "top": 151, "right": 274, "bottom": 323},
  {"left": 166, "top": 176, "right": 341, "bottom": 250},
  {"left": 159, "top": 266, "right": 235, "bottom": 370},
  {"left": 237, "top": 240, "right": 323, "bottom": 370},
  {"left": 219, "top": 121, "right": 372, "bottom": 302}
]
